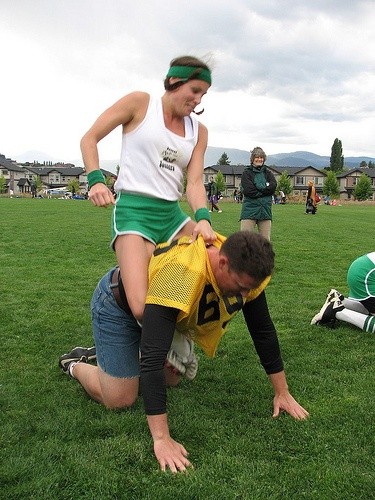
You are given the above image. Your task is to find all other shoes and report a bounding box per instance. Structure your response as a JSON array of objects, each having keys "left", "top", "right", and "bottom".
[
  {"left": 166, "top": 349, "right": 185, "bottom": 372},
  {"left": 183, "top": 338, "right": 198, "bottom": 380}
]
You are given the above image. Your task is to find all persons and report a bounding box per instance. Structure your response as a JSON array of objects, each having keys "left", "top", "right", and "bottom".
[
  {"left": 240, "top": 147, "right": 277, "bottom": 243},
  {"left": 304, "top": 180, "right": 317, "bottom": 215},
  {"left": 232, "top": 188, "right": 342, "bottom": 207},
  {"left": 311, "top": 252, "right": 375, "bottom": 333},
  {"left": 206, "top": 176, "right": 222, "bottom": 214},
  {"left": 58, "top": 231, "right": 307, "bottom": 472},
  {"left": 78, "top": 54, "right": 217, "bottom": 378},
  {"left": 5, "top": 184, "right": 117, "bottom": 202}
]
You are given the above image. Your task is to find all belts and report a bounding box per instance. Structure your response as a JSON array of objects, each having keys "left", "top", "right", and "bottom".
[{"left": 111, "top": 267, "right": 126, "bottom": 310}]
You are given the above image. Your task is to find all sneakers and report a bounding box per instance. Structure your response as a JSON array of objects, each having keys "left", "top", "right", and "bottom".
[
  {"left": 325, "top": 289, "right": 344, "bottom": 305},
  {"left": 58, "top": 346, "right": 88, "bottom": 376},
  {"left": 86, "top": 346, "right": 97, "bottom": 362},
  {"left": 310, "top": 297, "right": 345, "bottom": 327}
]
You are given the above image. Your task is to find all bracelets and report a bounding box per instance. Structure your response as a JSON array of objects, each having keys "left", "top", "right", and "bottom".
[
  {"left": 88, "top": 170, "right": 106, "bottom": 191},
  {"left": 195, "top": 208, "right": 212, "bottom": 225}
]
